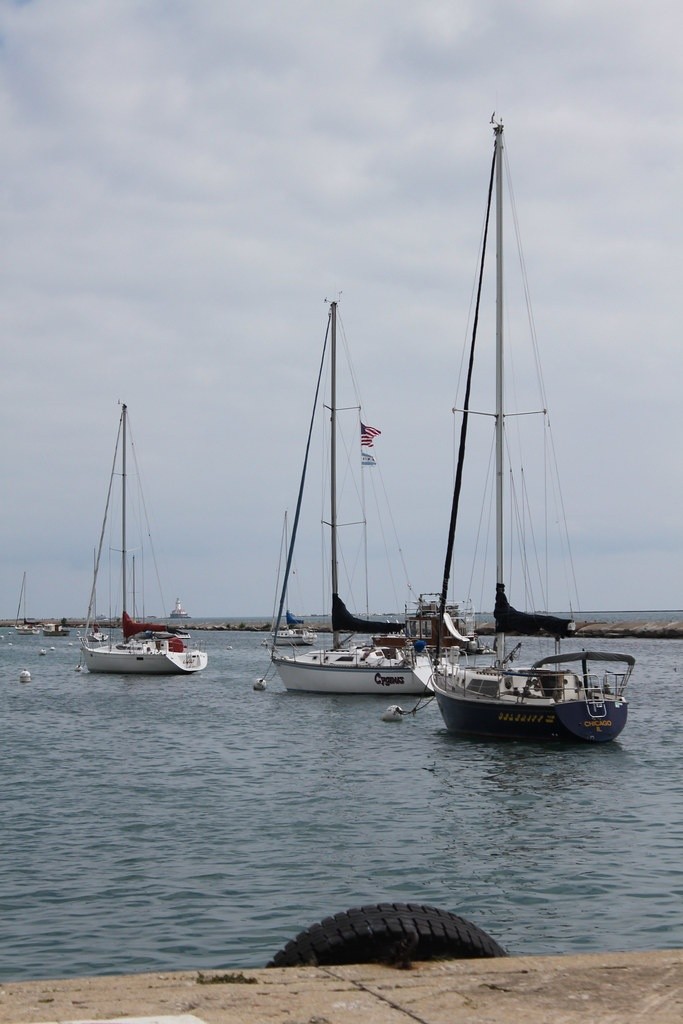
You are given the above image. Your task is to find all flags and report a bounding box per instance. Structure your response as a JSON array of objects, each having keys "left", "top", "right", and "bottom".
[
  {"left": 361, "top": 423, "right": 381, "bottom": 447},
  {"left": 362, "top": 452, "right": 376, "bottom": 466}
]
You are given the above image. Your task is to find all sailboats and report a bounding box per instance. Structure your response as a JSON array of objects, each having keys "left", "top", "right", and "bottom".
[
  {"left": 269, "top": 510, "right": 318, "bottom": 646},
  {"left": 270, "top": 291, "right": 435, "bottom": 696},
  {"left": 88, "top": 548, "right": 109, "bottom": 642},
  {"left": 14, "top": 572, "right": 40, "bottom": 635},
  {"left": 429, "top": 110, "right": 635, "bottom": 745},
  {"left": 78, "top": 398, "right": 207, "bottom": 675}
]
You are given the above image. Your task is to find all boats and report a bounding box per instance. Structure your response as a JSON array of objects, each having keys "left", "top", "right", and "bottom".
[
  {"left": 372, "top": 592, "right": 483, "bottom": 657},
  {"left": 42, "top": 624, "right": 70, "bottom": 636}
]
